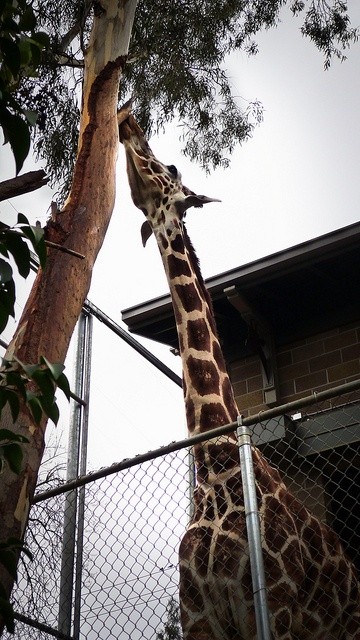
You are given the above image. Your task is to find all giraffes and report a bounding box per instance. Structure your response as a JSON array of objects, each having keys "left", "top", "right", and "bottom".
[{"left": 118, "top": 111, "right": 360, "bottom": 640}]
